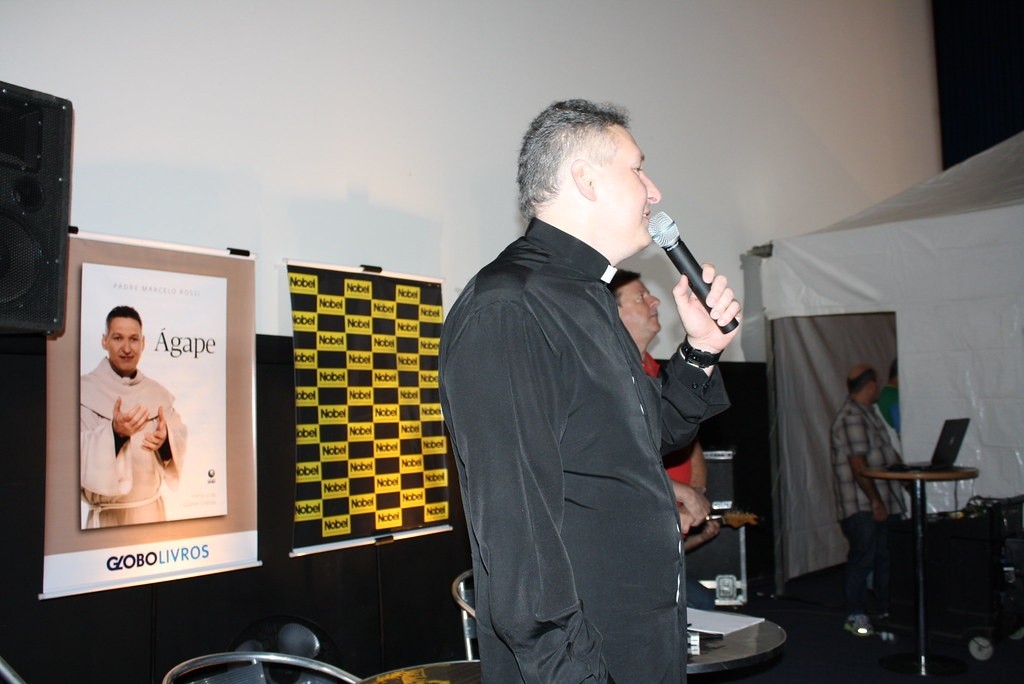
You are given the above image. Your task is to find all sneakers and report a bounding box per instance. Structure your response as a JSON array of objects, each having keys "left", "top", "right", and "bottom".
[{"left": 844, "top": 614, "right": 874, "bottom": 636}]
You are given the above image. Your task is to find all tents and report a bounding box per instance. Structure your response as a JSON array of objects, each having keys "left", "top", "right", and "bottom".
[{"left": 763, "top": 128, "right": 1023, "bottom": 595}]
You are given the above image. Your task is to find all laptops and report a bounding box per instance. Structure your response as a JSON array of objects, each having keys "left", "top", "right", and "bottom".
[{"left": 886, "top": 418, "right": 971, "bottom": 473}]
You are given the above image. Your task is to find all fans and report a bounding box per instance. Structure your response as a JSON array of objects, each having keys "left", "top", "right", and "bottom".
[{"left": 222, "top": 614, "right": 345, "bottom": 684}]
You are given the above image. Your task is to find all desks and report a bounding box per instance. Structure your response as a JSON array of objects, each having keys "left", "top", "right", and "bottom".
[
  {"left": 355, "top": 660, "right": 484, "bottom": 684},
  {"left": 860, "top": 461, "right": 981, "bottom": 677}
]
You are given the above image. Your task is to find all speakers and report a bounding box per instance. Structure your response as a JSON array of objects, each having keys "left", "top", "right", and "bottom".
[
  {"left": 702, "top": 449, "right": 741, "bottom": 513},
  {"left": 0, "top": 80, "right": 75, "bottom": 337}
]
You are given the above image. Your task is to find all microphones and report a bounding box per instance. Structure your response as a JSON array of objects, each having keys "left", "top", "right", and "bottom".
[{"left": 647, "top": 211, "right": 739, "bottom": 335}]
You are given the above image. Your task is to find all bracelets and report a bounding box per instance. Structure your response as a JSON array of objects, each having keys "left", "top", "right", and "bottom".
[{"left": 681, "top": 335, "right": 723, "bottom": 369}]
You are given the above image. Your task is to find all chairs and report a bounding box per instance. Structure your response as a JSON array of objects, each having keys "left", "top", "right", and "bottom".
[
  {"left": 161, "top": 651, "right": 364, "bottom": 684},
  {"left": 451, "top": 568, "right": 481, "bottom": 660}
]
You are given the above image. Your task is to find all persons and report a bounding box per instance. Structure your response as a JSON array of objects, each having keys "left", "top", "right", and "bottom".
[
  {"left": 876, "top": 357, "right": 899, "bottom": 439},
  {"left": 439, "top": 100, "right": 742, "bottom": 684},
  {"left": 80, "top": 306, "right": 187, "bottom": 529},
  {"left": 831, "top": 365, "right": 913, "bottom": 636},
  {"left": 606, "top": 269, "right": 720, "bottom": 611}
]
